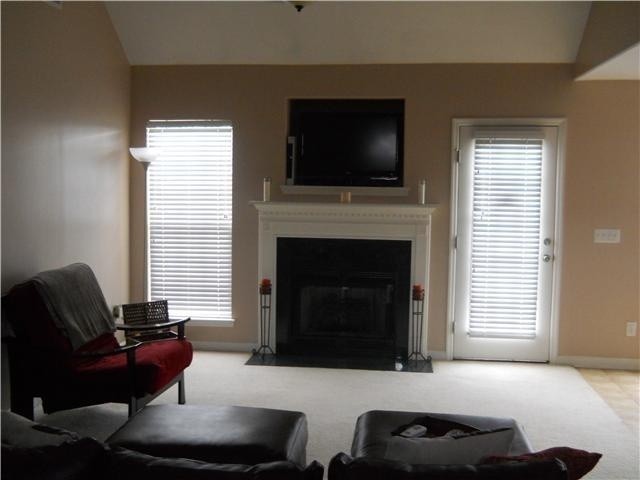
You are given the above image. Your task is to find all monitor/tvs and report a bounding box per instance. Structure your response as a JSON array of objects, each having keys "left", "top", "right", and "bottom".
[{"left": 285, "top": 97, "right": 406, "bottom": 188}]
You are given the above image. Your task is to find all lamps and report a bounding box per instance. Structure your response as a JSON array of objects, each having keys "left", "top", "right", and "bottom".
[{"left": 129, "top": 146, "right": 161, "bottom": 304}]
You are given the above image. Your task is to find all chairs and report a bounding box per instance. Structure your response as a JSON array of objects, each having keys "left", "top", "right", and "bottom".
[
  {"left": 326, "top": 410, "right": 601, "bottom": 478},
  {"left": 2, "top": 403, "right": 324, "bottom": 476},
  {"left": 1, "top": 263, "right": 194, "bottom": 420}
]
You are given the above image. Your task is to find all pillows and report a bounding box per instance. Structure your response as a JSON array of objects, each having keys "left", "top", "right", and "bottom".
[{"left": 387, "top": 427, "right": 515, "bottom": 463}]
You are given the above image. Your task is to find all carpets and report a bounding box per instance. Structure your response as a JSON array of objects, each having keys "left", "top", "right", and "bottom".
[{"left": 32, "top": 349, "right": 639, "bottom": 480}]
